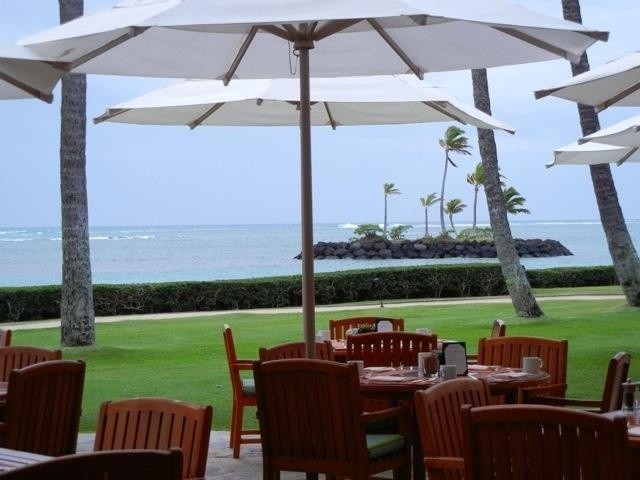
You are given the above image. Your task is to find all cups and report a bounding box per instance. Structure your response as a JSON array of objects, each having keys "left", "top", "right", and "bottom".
[
  {"left": 348, "top": 361, "right": 364, "bottom": 376},
  {"left": 418, "top": 352, "right": 432, "bottom": 371},
  {"left": 438, "top": 365, "right": 456, "bottom": 382},
  {"left": 319, "top": 330, "right": 330, "bottom": 341},
  {"left": 416, "top": 328, "right": 431, "bottom": 334},
  {"left": 523, "top": 356, "right": 543, "bottom": 374},
  {"left": 315, "top": 335, "right": 322, "bottom": 343}
]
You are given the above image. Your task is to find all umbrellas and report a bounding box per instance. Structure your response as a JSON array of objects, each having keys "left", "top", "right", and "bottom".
[
  {"left": 93, "top": 74, "right": 515, "bottom": 138},
  {"left": 576, "top": 112, "right": 640, "bottom": 148},
  {"left": 532, "top": 50, "right": 640, "bottom": 113},
  {"left": 1, "top": 40, "right": 65, "bottom": 103},
  {"left": 13, "top": 0, "right": 610, "bottom": 480},
  {"left": 545, "top": 138, "right": 640, "bottom": 167}
]
